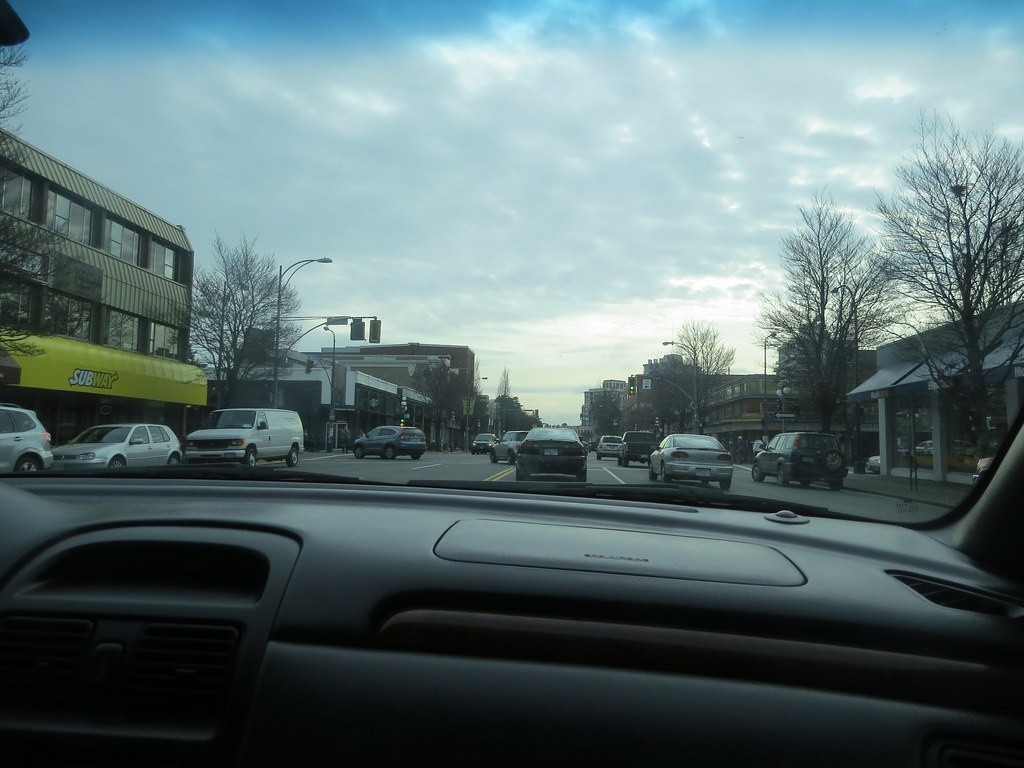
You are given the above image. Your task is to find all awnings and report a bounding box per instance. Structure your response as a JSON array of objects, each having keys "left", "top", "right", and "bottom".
[
  {"left": 846, "top": 337, "right": 1024, "bottom": 403},
  {"left": 1, "top": 330, "right": 208, "bottom": 406}
]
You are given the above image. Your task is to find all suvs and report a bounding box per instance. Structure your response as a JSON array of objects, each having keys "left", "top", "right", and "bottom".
[
  {"left": 0, "top": 403, "right": 53, "bottom": 473},
  {"left": 490, "top": 431, "right": 531, "bottom": 465},
  {"left": 972, "top": 457, "right": 993, "bottom": 478},
  {"left": 471, "top": 433, "right": 499, "bottom": 455},
  {"left": 597, "top": 435, "right": 623, "bottom": 460},
  {"left": 352, "top": 426, "right": 427, "bottom": 460},
  {"left": 588, "top": 441, "right": 598, "bottom": 453},
  {"left": 752, "top": 432, "right": 849, "bottom": 491}
]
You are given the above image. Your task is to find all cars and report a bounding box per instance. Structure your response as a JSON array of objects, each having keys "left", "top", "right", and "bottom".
[
  {"left": 516, "top": 427, "right": 587, "bottom": 482},
  {"left": 866, "top": 456, "right": 880, "bottom": 473},
  {"left": 916, "top": 441, "right": 933, "bottom": 455},
  {"left": 649, "top": 434, "right": 733, "bottom": 490}
]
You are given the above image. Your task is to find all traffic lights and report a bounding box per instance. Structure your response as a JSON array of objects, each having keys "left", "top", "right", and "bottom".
[{"left": 629, "top": 379, "right": 635, "bottom": 394}]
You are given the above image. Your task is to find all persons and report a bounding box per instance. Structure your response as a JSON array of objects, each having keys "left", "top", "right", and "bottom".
[
  {"left": 303, "top": 426, "right": 365, "bottom": 454},
  {"left": 721, "top": 435, "right": 763, "bottom": 465},
  {"left": 428, "top": 437, "right": 457, "bottom": 452}
]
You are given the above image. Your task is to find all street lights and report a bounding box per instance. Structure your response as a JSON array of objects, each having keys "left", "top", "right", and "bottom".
[
  {"left": 663, "top": 342, "right": 702, "bottom": 432},
  {"left": 274, "top": 256, "right": 334, "bottom": 451},
  {"left": 323, "top": 325, "right": 337, "bottom": 450},
  {"left": 830, "top": 285, "right": 865, "bottom": 474},
  {"left": 465, "top": 376, "right": 488, "bottom": 451},
  {"left": 763, "top": 333, "right": 791, "bottom": 436}
]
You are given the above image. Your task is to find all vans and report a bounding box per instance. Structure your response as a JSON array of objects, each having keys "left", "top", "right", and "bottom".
[
  {"left": 51, "top": 424, "right": 182, "bottom": 470},
  {"left": 185, "top": 408, "right": 304, "bottom": 467}
]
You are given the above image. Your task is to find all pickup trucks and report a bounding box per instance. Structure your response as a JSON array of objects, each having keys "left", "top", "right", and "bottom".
[{"left": 617, "top": 431, "right": 661, "bottom": 467}]
